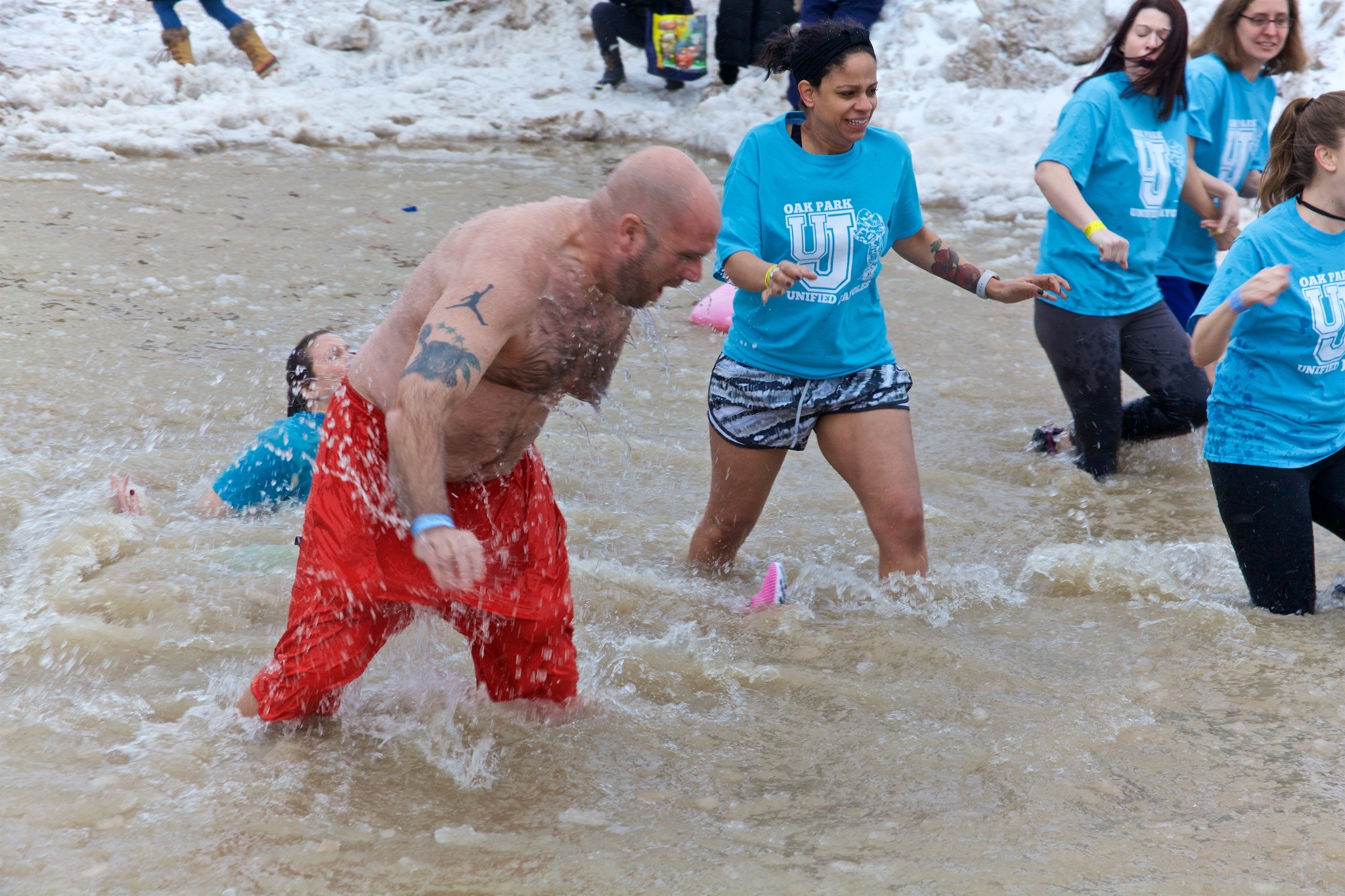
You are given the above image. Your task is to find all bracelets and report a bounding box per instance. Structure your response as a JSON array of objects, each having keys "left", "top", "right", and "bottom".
[
  {"left": 410, "top": 513, "right": 456, "bottom": 544},
  {"left": 764, "top": 263, "right": 780, "bottom": 287},
  {"left": 1081, "top": 220, "right": 1106, "bottom": 238},
  {"left": 976, "top": 268, "right": 1001, "bottom": 300},
  {"left": 1227, "top": 290, "right": 1251, "bottom": 313}
]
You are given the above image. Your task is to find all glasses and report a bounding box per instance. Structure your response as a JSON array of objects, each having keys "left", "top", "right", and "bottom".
[{"left": 1240, "top": 13, "right": 1294, "bottom": 28}]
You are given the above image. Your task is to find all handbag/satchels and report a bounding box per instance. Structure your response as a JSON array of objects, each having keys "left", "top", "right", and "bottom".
[{"left": 644, "top": 2, "right": 707, "bottom": 80}]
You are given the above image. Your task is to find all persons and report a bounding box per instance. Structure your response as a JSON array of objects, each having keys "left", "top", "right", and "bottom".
[
  {"left": 677, "top": 17, "right": 1076, "bottom": 613},
  {"left": 1152, "top": 0, "right": 1310, "bottom": 342},
  {"left": 787, "top": 1, "right": 880, "bottom": 47},
  {"left": 234, "top": 142, "right": 728, "bottom": 730},
  {"left": 588, "top": 1, "right": 701, "bottom": 89},
  {"left": 1187, "top": 91, "right": 1344, "bottom": 615},
  {"left": 108, "top": 325, "right": 360, "bottom": 520},
  {"left": 1030, "top": 0, "right": 1239, "bottom": 478},
  {"left": 147, "top": 0, "right": 281, "bottom": 80},
  {"left": 699, "top": 0, "right": 801, "bottom": 95}
]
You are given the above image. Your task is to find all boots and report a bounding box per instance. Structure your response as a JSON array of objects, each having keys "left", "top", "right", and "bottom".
[
  {"left": 657, "top": 77, "right": 685, "bottom": 90},
  {"left": 229, "top": 19, "right": 280, "bottom": 76},
  {"left": 596, "top": 65, "right": 625, "bottom": 89},
  {"left": 163, "top": 25, "right": 198, "bottom": 67}
]
